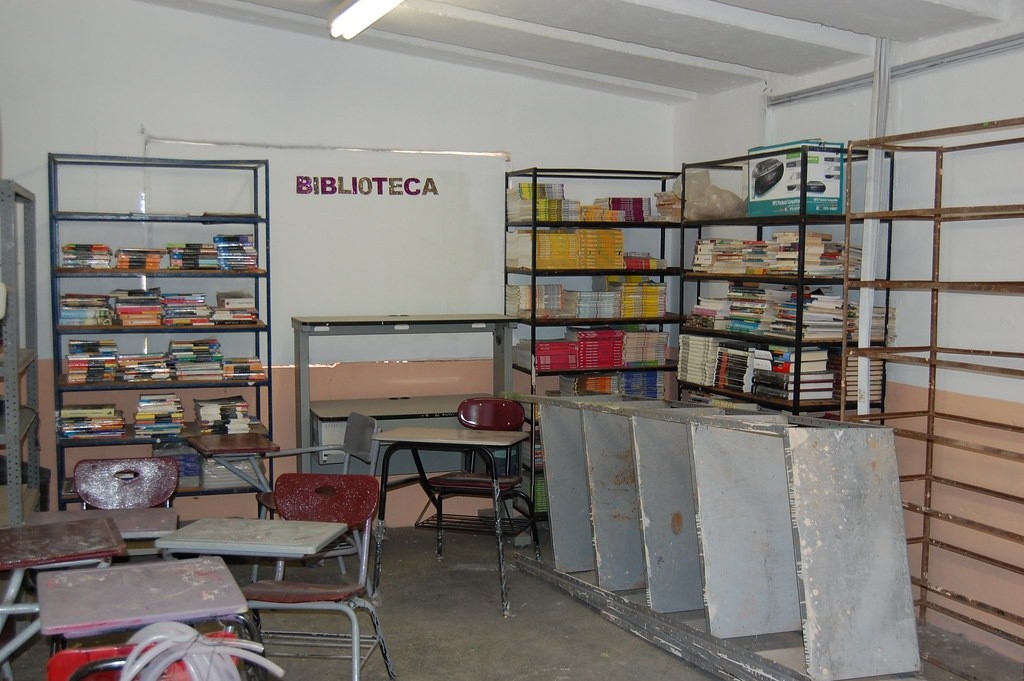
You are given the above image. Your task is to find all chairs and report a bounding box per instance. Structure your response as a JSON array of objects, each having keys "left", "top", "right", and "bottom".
[
  {"left": 71, "top": 456, "right": 183, "bottom": 568},
  {"left": 251, "top": 411, "right": 383, "bottom": 583},
  {"left": 244, "top": 470, "right": 401, "bottom": 680},
  {"left": 46, "top": 631, "right": 248, "bottom": 680},
  {"left": 422, "top": 393, "right": 545, "bottom": 566}
]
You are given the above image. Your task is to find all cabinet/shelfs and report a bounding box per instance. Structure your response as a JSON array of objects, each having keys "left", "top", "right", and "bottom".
[
  {"left": 0, "top": 178, "right": 43, "bottom": 530},
  {"left": 289, "top": 307, "right": 524, "bottom": 472},
  {"left": 47, "top": 152, "right": 277, "bottom": 522},
  {"left": 503, "top": 115, "right": 1023, "bottom": 681}
]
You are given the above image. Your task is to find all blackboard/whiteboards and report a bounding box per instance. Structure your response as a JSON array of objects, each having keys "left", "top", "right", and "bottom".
[{"left": 136, "top": 133, "right": 510, "bottom": 371}]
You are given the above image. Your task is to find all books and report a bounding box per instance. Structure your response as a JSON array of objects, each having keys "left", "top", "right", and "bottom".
[
  {"left": 56, "top": 232, "right": 268, "bottom": 441},
  {"left": 506, "top": 179, "right": 896, "bottom": 512}
]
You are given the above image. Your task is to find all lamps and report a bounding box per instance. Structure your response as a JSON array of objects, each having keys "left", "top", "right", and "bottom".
[{"left": 327, "top": 0, "right": 409, "bottom": 42}]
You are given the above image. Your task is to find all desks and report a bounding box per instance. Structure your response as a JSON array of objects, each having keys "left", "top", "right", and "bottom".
[
  {"left": 185, "top": 431, "right": 280, "bottom": 504},
  {"left": 151, "top": 517, "right": 350, "bottom": 561},
  {"left": 1, "top": 515, "right": 128, "bottom": 681},
  {"left": 33, "top": 555, "right": 251, "bottom": 655},
  {"left": 20, "top": 508, "right": 180, "bottom": 540},
  {"left": 365, "top": 425, "right": 532, "bottom": 620}
]
